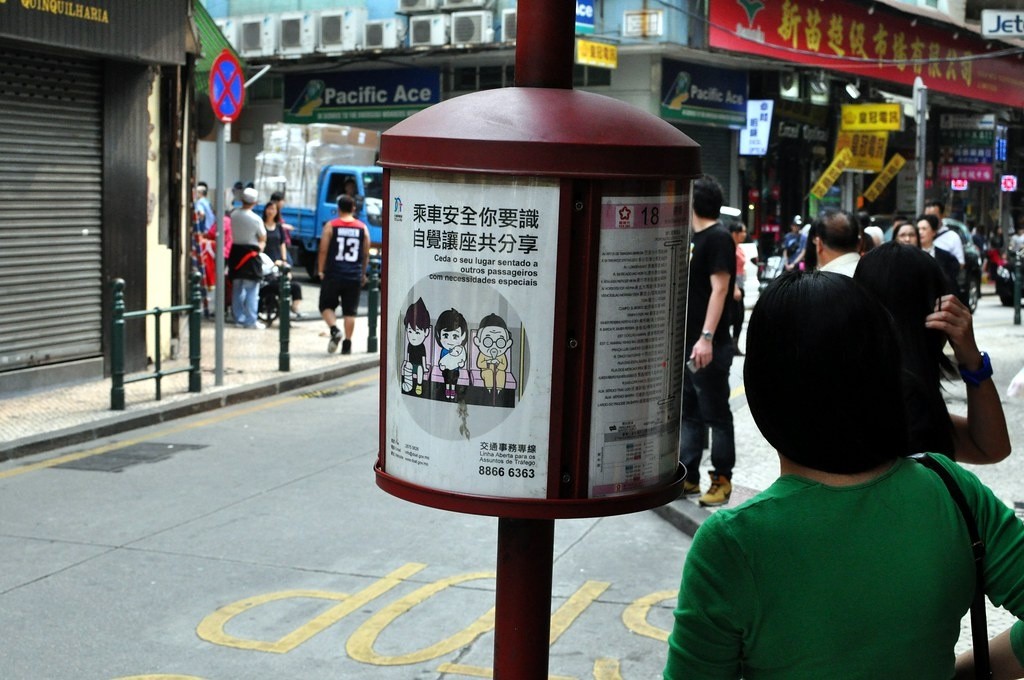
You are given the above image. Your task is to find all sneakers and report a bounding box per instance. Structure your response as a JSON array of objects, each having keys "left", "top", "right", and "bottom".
[
  {"left": 697, "top": 470, "right": 733, "bottom": 509},
  {"left": 681, "top": 479, "right": 702, "bottom": 499}
]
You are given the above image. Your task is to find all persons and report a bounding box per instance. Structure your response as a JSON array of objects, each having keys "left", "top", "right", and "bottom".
[
  {"left": 679, "top": 174, "right": 735, "bottom": 506},
  {"left": 728, "top": 222, "right": 747, "bottom": 356},
  {"left": 853, "top": 239, "right": 1012, "bottom": 466},
  {"left": 318, "top": 194, "right": 370, "bottom": 354},
  {"left": 663, "top": 268, "right": 1024, "bottom": 680},
  {"left": 336, "top": 179, "right": 356, "bottom": 204},
  {"left": 262, "top": 202, "right": 302, "bottom": 318},
  {"left": 812, "top": 210, "right": 862, "bottom": 279},
  {"left": 230, "top": 188, "right": 267, "bottom": 329},
  {"left": 271, "top": 192, "right": 296, "bottom": 265},
  {"left": 779, "top": 200, "right": 1024, "bottom": 378},
  {"left": 192, "top": 181, "right": 254, "bottom": 233}
]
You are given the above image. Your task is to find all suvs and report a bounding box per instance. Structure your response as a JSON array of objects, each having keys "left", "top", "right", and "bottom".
[{"left": 867, "top": 212, "right": 983, "bottom": 314}]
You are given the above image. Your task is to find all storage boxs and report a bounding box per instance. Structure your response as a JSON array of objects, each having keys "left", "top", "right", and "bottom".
[{"left": 307, "top": 123, "right": 382, "bottom": 151}]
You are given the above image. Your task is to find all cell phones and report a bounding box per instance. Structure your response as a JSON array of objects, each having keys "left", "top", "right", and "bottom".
[{"left": 687, "top": 358, "right": 699, "bottom": 374}]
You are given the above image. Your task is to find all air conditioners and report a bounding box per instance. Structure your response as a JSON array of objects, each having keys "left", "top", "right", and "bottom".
[
  {"left": 450, "top": 10, "right": 495, "bottom": 46},
  {"left": 501, "top": 8, "right": 516, "bottom": 44},
  {"left": 277, "top": 11, "right": 319, "bottom": 56},
  {"left": 396, "top": 0, "right": 438, "bottom": 15},
  {"left": 211, "top": 16, "right": 241, "bottom": 54},
  {"left": 804, "top": 73, "right": 831, "bottom": 105},
  {"left": 318, "top": 8, "right": 368, "bottom": 53},
  {"left": 760, "top": 68, "right": 799, "bottom": 100},
  {"left": 409, "top": 14, "right": 451, "bottom": 47},
  {"left": 241, "top": 13, "right": 278, "bottom": 58},
  {"left": 362, "top": 19, "right": 406, "bottom": 49},
  {"left": 438, "top": 0, "right": 486, "bottom": 10}
]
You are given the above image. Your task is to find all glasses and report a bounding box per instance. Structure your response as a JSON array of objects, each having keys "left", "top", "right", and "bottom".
[{"left": 895, "top": 232, "right": 922, "bottom": 238}]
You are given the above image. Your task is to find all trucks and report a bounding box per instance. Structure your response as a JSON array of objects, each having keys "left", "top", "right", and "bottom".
[{"left": 234, "top": 164, "right": 383, "bottom": 291}]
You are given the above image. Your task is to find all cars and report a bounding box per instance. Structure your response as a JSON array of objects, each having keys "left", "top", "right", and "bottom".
[{"left": 994, "top": 244, "right": 1024, "bottom": 307}]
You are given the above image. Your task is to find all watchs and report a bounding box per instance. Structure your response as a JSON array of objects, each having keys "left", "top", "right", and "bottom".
[
  {"left": 701, "top": 331, "right": 712, "bottom": 340},
  {"left": 958, "top": 352, "right": 993, "bottom": 387}
]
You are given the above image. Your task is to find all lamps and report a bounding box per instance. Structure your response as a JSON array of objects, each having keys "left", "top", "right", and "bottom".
[
  {"left": 810, "top": 70, "right": 828, "bottom": 96},
  {"left": 845, "top": 74, "right": 862, "bottom": 99}
]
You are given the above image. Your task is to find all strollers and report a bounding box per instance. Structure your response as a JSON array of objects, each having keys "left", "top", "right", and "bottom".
[{"left": 257, "top": 252, "right": 304, "bottom": 327}]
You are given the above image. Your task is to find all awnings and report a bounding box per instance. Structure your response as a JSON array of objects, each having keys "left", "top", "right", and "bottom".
[{"left": 192, "top": 0, "right": 247, "bottom": 92}]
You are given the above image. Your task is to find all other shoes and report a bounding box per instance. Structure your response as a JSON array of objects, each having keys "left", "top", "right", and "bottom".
[
  {"left": 288, "top": 307, "right": 311, "bottom": 320},
  {"left": 249, "top": 321, "right": 267, "bottom": 331},
  {"left": 327, "top": 326, "right": 342, "bottom": 355},
  {"left": 341, "top": 338, "right": 352, "bottom": 355}
]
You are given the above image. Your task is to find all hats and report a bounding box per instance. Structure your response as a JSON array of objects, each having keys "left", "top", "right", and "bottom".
[
  {"left": 241, "top": 187, "right": 259, "bottom": 204},
  {"left": 792, "top": 215, "right": 806, "bottom": 226}
]
